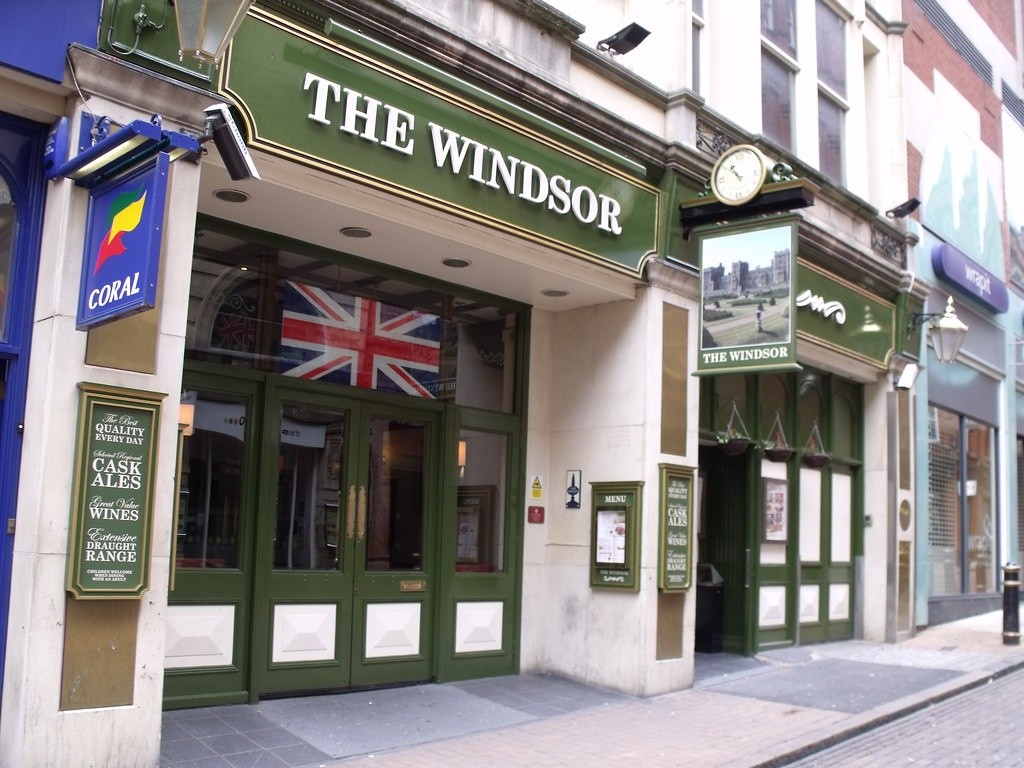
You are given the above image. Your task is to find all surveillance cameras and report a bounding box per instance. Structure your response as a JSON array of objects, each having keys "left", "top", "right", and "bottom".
[
  {"left": 202, "top": 103, "right": 261, "bottom": 182},
  {"left": 897, "top": 364, "right": 918, "bottom": 391}
]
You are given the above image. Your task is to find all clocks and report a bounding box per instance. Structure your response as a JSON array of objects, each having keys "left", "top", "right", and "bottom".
[{"left": 710, "top": 144, "right": 766, "bottom": 206}]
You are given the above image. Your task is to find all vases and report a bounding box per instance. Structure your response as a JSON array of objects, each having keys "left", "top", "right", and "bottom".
[
  {"left": 801, "top": 454, "right": 831, "bottom": 468},
  {"left": 720, "top": 439, "right": 751, "bottom": 455},
  {"left": 765, "top": 449, "right": 794, "bottom": 462}
]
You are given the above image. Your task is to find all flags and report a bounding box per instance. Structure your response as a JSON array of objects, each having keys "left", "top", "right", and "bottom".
[{"left": 276, "top": 281, "right": 443, "bottom": 400}]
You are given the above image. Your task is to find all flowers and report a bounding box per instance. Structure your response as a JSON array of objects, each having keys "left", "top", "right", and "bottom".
[
  {"left": 804, "top": 438, "right": 832, "bottom": 456},
  {"left": 714, "top": 423, "right": 744, "bottom": 444},
  {"left": 762, "top": 431, "right": 786, "bottom": 449}
]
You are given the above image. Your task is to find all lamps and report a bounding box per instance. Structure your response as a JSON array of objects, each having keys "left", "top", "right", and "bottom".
[
  {"left": 597, "top": 22, "right": 651, "bottom": 58},
  {"left": 93, "top": 131, "right": 199, "bottom": 191},
  {"left": 58, "top": 113, "right": 162, "bottom": 183},
  {"left": 906, "top": 295, "right": 970, "bottom": 365},
  {"left": 167, "top": 0, "right": 255, "bottom": 70},
  {"left": 886, "top": 198, "right": 921, "bottom": 218}
]
